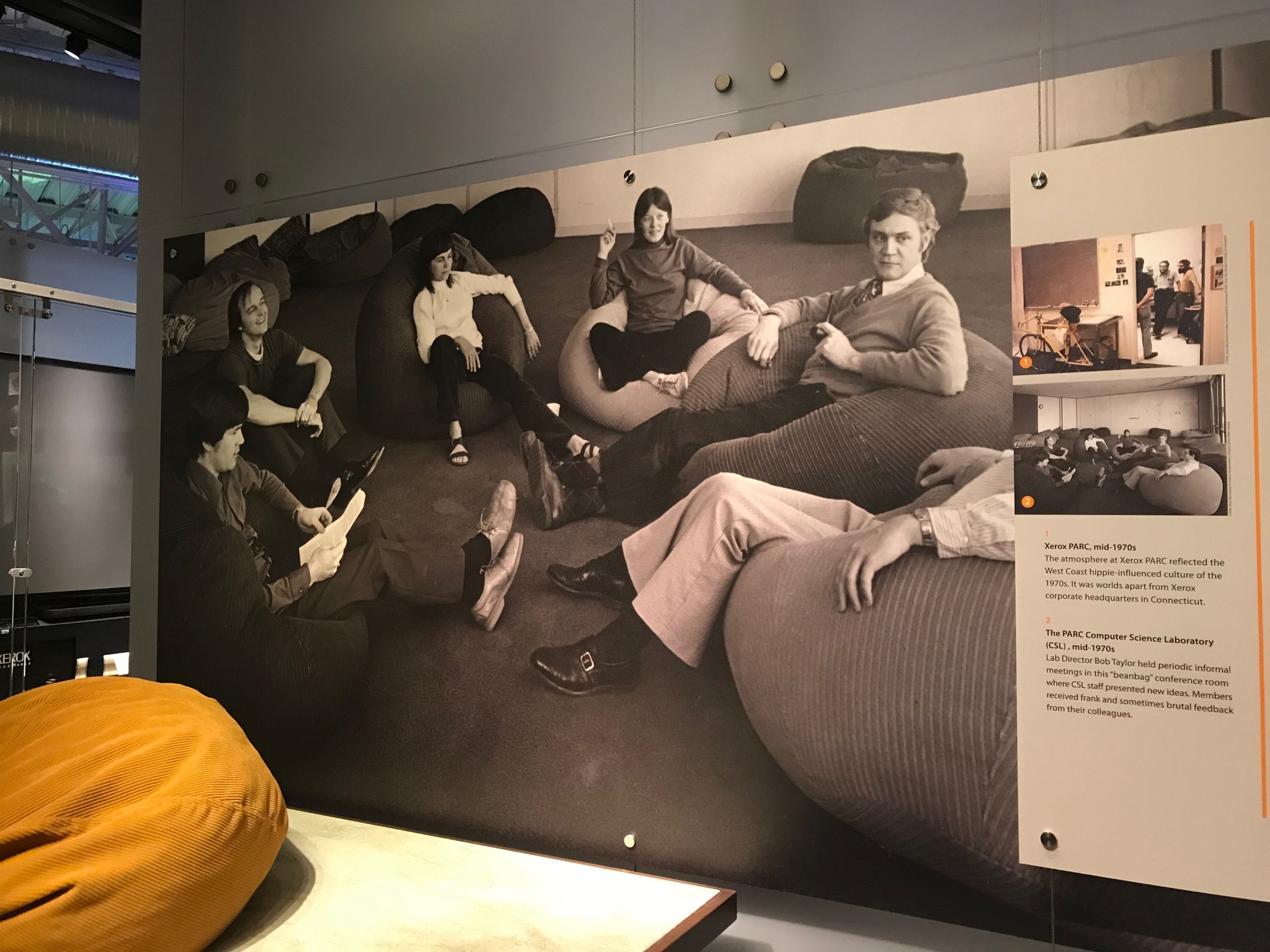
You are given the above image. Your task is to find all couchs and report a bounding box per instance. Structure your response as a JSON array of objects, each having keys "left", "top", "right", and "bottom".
[{"left": 156, "top": 110, "right": 1269, "bottom": 935}]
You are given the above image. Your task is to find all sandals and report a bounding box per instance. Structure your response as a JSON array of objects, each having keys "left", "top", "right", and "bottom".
[
  {"left": 572, "top": 440, "right": 606, "bottom": 461},
  {"left": 449, "top": 435, "right": 468, "bottom": 466}
]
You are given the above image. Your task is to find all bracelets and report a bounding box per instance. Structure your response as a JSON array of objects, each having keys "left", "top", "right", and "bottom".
[
  {"left": 294, "top": 504, "right": 304, "bottom": 527},
  {"left": 524, "top": 327, "right": 534, "bottom": 335},
  {"left": 306, "top": 395, "right": 319, "bottom": 402}
]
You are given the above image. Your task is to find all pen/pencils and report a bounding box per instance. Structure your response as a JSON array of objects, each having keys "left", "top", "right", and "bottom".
[{"left": 608, "top": 217, "right": 613, "bottom": 228}]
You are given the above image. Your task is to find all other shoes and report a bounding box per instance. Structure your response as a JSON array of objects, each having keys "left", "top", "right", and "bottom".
[
  {"left": 469, "top": 532, "right": 525, "bottom": 632},
  {"left": 598, "top": 368, "right": 604, "bottom": 389},
  {"left": 474, "top": 480, "right": 515, "bottom": 563},
  {"left": 657, "top": 372, "right": 688, "bottom": 398},
  {"left": 527, "top": 440, "right": 568, "bottom": 530},
  {"left": 520, "top": 431, "right": 536, "bottom": 473}
]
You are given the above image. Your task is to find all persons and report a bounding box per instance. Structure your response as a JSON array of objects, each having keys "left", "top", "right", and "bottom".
[
  {"left": 182, "top": 376, "right": 525, "bottom": 632},
  {"left": 215, "top": 281, "right": 386, "bottom": 509},
  {"left": 1135, "top": 258, "right": 1201, "bottom": 359},
  {"left": 522, "top": 188, "right": 968, "bottom": 530},
  {"left": 531, "top": 447, "right": 1014, "bottom": 694},
  {"left": 1034, "top": 430, "right": 1201, "bottom": 494},
  {"left": 413, "top": 231, "right": 608, "bottom": 466},
  {"left": 589, "top": 187, "right": 768, "bottom": 399}
]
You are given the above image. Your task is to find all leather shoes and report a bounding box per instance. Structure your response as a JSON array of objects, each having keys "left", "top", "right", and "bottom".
[
  {"left": 530, "top": 636, "right": 638, "bottom": 695},
  {"left": 322, "top": 476, "right": 341, "bottom": 510},
  {"left": 336, "top": 446, "right": 385, "bottom": 496},
  {"left": 545, "top": 557, "right": 638, "bottom": 612}
]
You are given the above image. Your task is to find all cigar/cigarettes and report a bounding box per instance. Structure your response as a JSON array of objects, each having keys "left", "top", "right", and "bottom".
[{"left": 608, "top": 217, "right": 613, "bottom": 230}]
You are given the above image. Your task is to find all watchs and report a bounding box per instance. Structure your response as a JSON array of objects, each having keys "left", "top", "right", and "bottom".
[{"left": 913, "top": 508, "right": 933, "bottom": 547}]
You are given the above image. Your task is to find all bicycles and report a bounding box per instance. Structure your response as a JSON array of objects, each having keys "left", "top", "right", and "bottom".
[{"left": 1017, "top": 303, "right": 1121, "bottom": 371}]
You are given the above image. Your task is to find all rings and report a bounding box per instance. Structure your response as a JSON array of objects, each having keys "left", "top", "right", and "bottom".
[
  {"left": 469, "top": 356, "right": 472, "bottom": 357},
  {"left": 536, "top": 348, "right": 538, "bottom": 350},
  {"left": 337, "top": 563, "right": 340, "bottom": 567}
]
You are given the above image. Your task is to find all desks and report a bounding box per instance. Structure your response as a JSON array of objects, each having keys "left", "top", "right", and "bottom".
[{"left": 205, "top": 807, "right": 739, "bottom": 952}]
